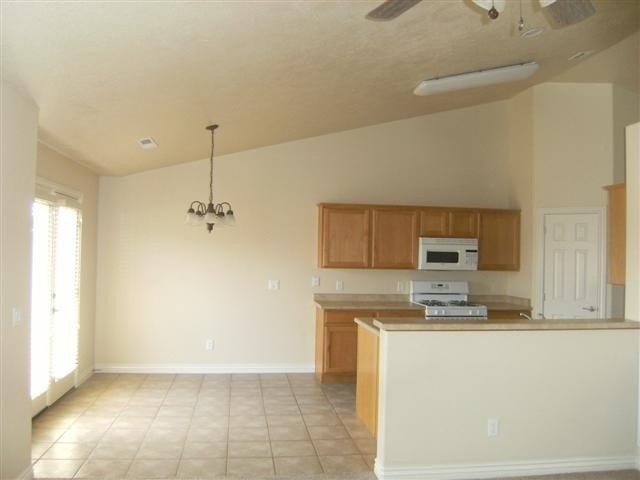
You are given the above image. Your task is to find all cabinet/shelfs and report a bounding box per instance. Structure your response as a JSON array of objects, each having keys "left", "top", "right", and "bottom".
[
  {"left": 316, "top": 308, "right": 531, "bottom": 383},
  {"left": 601, "top": 181, "right": 626, "bottom": 287},
  {"left": 318, "top": 199, "right": 518, "bottom": 271}
]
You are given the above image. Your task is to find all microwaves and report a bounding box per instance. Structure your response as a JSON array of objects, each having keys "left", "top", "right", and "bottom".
[{"left": 417, "top": 237, "right": 480, "bottom": 272}]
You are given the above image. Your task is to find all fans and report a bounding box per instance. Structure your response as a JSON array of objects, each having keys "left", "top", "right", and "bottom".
[{"left": 365, "top": 0, "right": 596, "bottom": 29}]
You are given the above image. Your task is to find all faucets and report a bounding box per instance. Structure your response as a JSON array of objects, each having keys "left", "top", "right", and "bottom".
[{"left": 519, "top": 312, "right": 532, "bottom": 320}]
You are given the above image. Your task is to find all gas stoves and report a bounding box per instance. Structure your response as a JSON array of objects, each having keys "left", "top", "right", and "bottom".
[{"left": 416, "top": 299, "right": 486, "bottom": 309}]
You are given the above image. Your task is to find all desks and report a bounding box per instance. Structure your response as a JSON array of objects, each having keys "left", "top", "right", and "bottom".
[{"left": 354, "top": 317, "right": 379, "bottom": 440}]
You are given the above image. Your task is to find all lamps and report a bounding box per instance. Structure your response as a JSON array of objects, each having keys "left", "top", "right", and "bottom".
[
  {"left": 413, "top": 61, "right": 540, "bottom": 96},
  {"left": 185, "top": 124, "right": 236, "bottom": 234}
]
